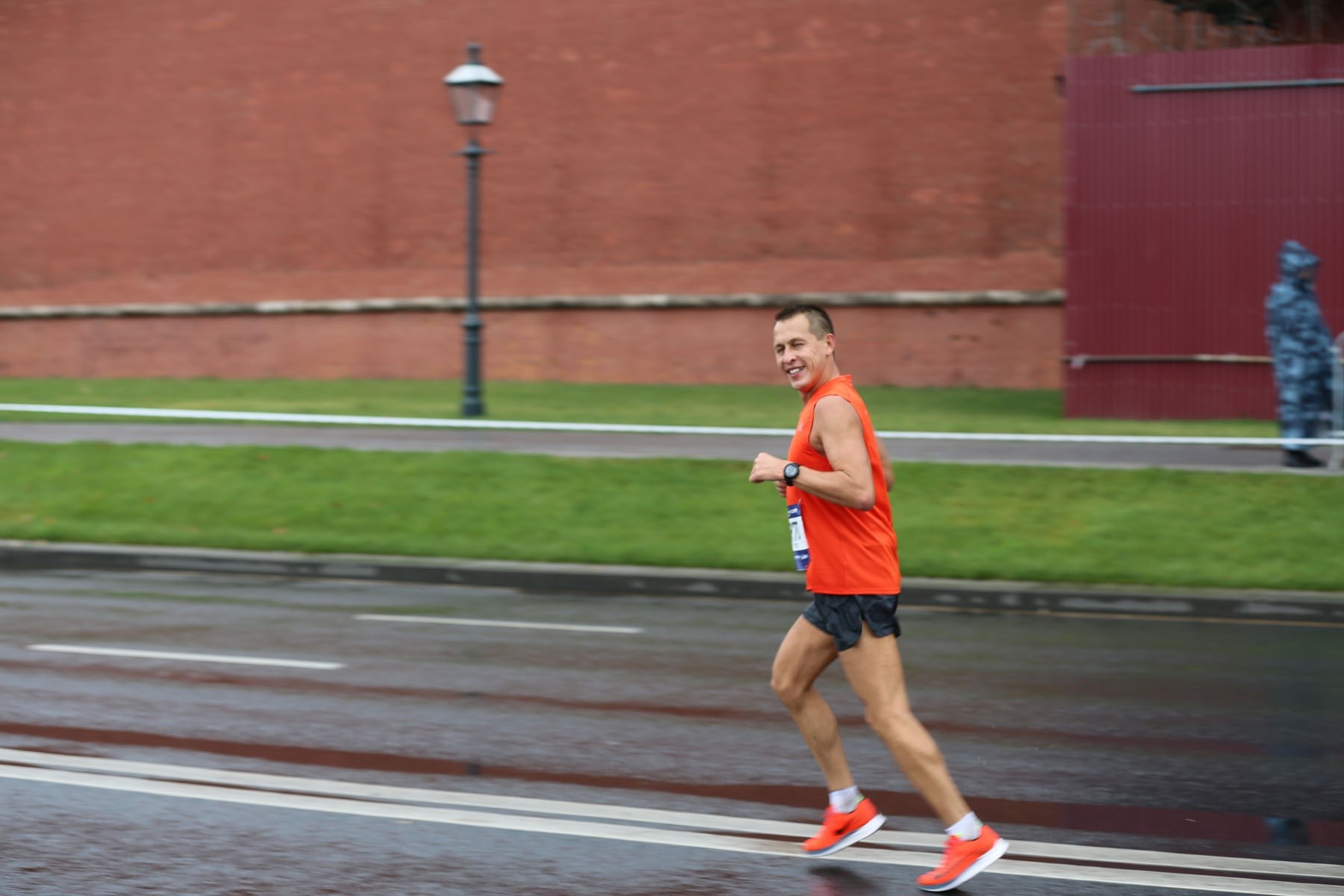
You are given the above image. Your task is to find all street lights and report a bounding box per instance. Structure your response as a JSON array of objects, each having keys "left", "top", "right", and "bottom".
[{"left": 441, "top": 43, "right": 505, "bottom": 418}]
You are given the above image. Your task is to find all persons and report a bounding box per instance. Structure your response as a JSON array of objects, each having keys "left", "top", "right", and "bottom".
[
  {"left": 751, "top": 303, "right": 1008, "bottom": 893},
  {"left": 1267, "top": 241, "right": 1334, "bottom": 468}
]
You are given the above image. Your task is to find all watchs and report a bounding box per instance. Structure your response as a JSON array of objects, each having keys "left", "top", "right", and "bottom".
[{"left": 784, "top": 463, "right": 803, "bottom": 486}]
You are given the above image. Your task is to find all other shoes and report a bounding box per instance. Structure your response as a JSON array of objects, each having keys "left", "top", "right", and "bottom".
[{"left": 1280, "top": 449, "right": 1325, "bottom": 469}]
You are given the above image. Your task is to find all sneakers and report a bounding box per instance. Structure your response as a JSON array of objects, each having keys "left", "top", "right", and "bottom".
[
  {"left": 917, "top": 825, "right": 1008, "bottom": 892},
  {"left": 802, "top": 799, "right": 886, "bottom": 857}
]
